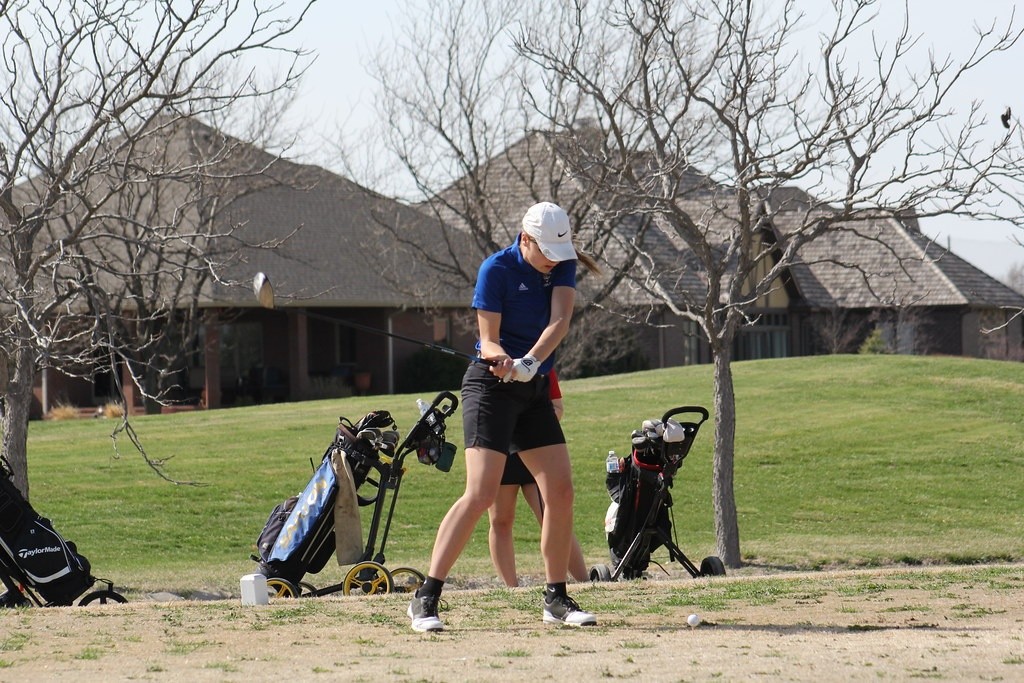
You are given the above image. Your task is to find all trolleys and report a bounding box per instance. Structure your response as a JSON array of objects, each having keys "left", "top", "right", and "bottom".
[
  {"left": 590, "top": 406, "right": 727, "bottom": 582},
  {"left": 0, "top": 455, "right": 129, "bottom": 610},
  {"left": 250, "top": 390, "right": 461, "bottom": 596}
]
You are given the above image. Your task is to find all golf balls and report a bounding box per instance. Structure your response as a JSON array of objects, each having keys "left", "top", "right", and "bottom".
[{"left": 688, "top": 614, "right": 699, "bottom": 627}]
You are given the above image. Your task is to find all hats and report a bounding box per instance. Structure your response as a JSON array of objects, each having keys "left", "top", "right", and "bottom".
[{"left": 522, "top": 201, "right": 578, "bottom": 262}]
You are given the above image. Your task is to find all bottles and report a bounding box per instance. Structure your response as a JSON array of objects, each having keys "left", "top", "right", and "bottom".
[
  {"left": 416, "top": 397, "right": 442, "bottom": 434},
  {"left": 606, "top": 450, "right": 619, "bottom": 474}
]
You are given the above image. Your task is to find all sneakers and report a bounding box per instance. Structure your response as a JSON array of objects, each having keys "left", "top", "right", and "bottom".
[
  {"left": 407, "top": 589, "right": 449, "bottom": 632},
  {"left": 542, "top": 591, "right": 598, "bottom": 626}
]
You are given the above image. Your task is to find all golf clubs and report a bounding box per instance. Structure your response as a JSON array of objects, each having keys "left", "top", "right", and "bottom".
[
  {"left": 252, "top": 271, "right": 499, "bottom": 367},
  {"left": 356, "top": 427, "right": 400, "bottom": 457},
  {"left": 632, "top": 418, "right": 665, "bottom": 464}
]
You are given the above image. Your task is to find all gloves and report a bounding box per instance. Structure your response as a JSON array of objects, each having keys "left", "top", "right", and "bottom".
[{"left": 503, "top": 354, "right": 542, "bottom": 383}]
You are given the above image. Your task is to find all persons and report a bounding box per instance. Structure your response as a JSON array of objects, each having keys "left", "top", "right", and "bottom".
[
  {"left": 406, "top": 201, "right": 598, "bottom": 635},
  {"left": 485, "top": 363, "right": 593, "bottom": 589}
]
